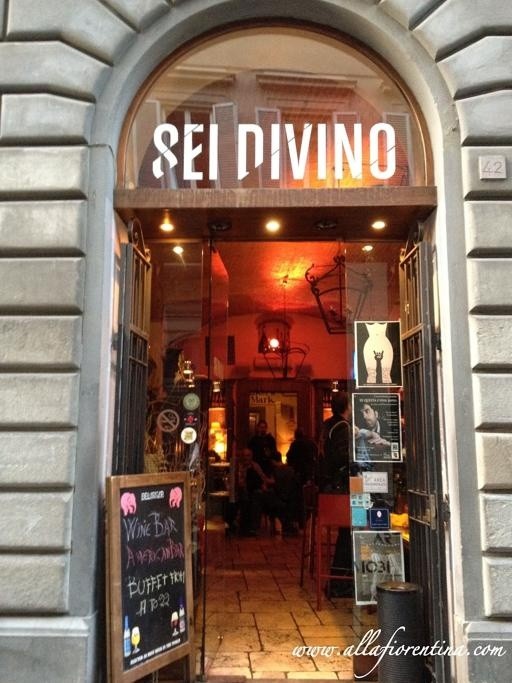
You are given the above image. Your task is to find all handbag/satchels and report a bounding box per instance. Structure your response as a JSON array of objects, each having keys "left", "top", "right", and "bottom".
[{"left": 326, "top": 470, "right": 346, "bottom": 494}]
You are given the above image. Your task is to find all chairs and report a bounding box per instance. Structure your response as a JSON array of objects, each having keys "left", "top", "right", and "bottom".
[
  {"left": 314, "top": 495, "right": 350, "bottom": 612},
  {"left": 300, "top": 480, "right": 335, "bottom": 588}
]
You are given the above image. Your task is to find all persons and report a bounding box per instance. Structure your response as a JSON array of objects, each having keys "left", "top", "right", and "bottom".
[
  {"left": 236, "top": 391, "right": 350, "bottom": 538},
  {"left": 355, "top": 401, "right": 397, "bottom": 456}
]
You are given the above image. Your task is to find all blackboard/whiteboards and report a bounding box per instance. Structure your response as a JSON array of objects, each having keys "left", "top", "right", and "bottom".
[{"left": 105, "top": 471, "right": 194, "bottom": 683}]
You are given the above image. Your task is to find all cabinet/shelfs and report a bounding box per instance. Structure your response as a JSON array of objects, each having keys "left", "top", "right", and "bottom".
[{"left": 209, "top": 461, "right": 231, "bottom": 497}]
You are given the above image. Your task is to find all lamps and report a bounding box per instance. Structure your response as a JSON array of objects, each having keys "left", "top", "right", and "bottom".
[
  {"left": 307, "top": 251, "right": 372, "bottom": 334},
  {"left": 261, "top": 279, "right": 309, "bottom": 382}
]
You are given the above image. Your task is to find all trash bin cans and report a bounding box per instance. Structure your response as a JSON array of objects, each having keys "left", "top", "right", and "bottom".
[{"left": 375, "top": 580, "right": 423, "bottom": 683}]
